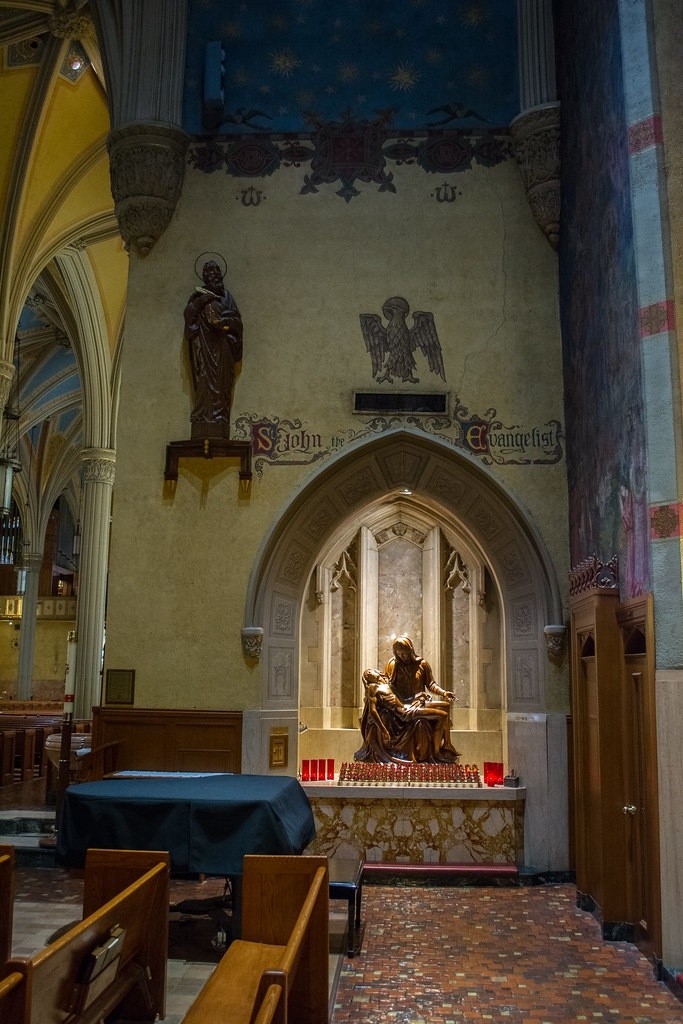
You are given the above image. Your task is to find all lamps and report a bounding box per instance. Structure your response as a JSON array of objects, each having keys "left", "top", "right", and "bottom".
[
  {"left": 72, "top": 519, "right": 81, "bottom": 557},
  {"left": 0, "top": 321, "right": 23, "bottom": 517},
  {"left": 67, "top": 53, "right": 83, "bottom": 69}
]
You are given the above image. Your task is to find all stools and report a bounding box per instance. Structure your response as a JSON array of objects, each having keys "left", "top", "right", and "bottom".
[{"left": 328, "top": 859, "right": 365, "bottom": 958}]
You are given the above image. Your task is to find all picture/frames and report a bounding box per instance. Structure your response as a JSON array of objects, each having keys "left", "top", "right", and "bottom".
[
  {"left": 105, "top": 669, "right": 135, "bottom": 704},
  {"left": 270, "top": 734, "right": 289, "bottom": 768}
]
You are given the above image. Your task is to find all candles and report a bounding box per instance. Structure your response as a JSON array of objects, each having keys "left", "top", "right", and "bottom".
[
  {"left": 327, "top": 759, "right": 334, "bottom": 780},
  {"left": 484, "top": 762, "right": 503, "bottom": 787},
  {"left": 310, "top": 760, "right": 318, "bottom": 781},
  {"left": 340, "top": 761, "right": 479, "bottom": 783},
  {"left": 319, "top": 758, "right": 326, "bottom": 780},
  {"left": 302, "top": 760, "right": 310, "bottom": 781}
]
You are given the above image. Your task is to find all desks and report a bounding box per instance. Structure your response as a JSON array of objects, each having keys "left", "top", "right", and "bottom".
[
  {"left": 103, "top": 770, "right": 234, "bottom": 780},
  {"left": 57, "top": 775, "right": 318, "bottom": 915}
]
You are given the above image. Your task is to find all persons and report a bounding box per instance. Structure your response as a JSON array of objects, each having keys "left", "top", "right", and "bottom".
[
  {"left": 183, "top": 260, "right": 244, "bottom": 442},
  {"left": 382, "top": 636, "right": 457, "bottom": 703},
  {"left": 362, "top": 668, "right": 460, "bottom": 764}
]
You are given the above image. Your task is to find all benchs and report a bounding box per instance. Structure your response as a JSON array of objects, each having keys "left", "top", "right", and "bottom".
[
  {"left": 180, "top": 854, "right": 329, "bottom": 1024},
  {"left": 0, "top": 714, "right": 62, "bottom": 787},
  {"left": 0, "top": 849, "right": 171, "bottom": 1024}
]
